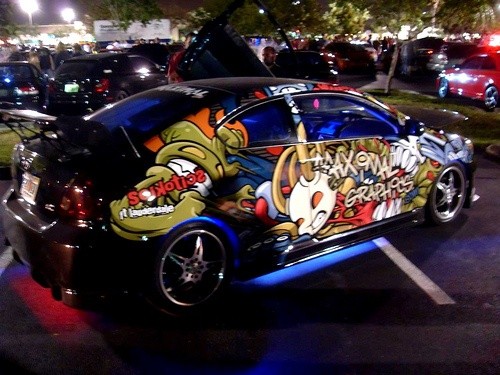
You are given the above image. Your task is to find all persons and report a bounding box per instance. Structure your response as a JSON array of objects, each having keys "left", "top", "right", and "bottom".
[
  {"left": 261, "top": 44, "right": 290, "bottom": 79},
  {"left": 247, "top": 34, "right": 481, "bottom": 56},
  {"left": 169, "top": 32, "right": 197, "bottom": 83},
  {"left": 0, "top": 37, "right": 167, "bottom": 73}
]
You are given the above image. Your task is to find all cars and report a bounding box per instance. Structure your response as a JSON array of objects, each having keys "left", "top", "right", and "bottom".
[
  {"left": 0, "top": 0, "right": 476, "bottom": 318},
  {"left": 0, "top": 61, "right": 49, "bottom": 111},
  {"left": 436, "top": 53, "right": 500, "bottom": 108}
]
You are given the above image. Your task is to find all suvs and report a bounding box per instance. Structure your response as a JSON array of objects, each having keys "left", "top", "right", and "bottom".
[{"left": 49, "top": 52, "right": 168, "bottom": 112}]
